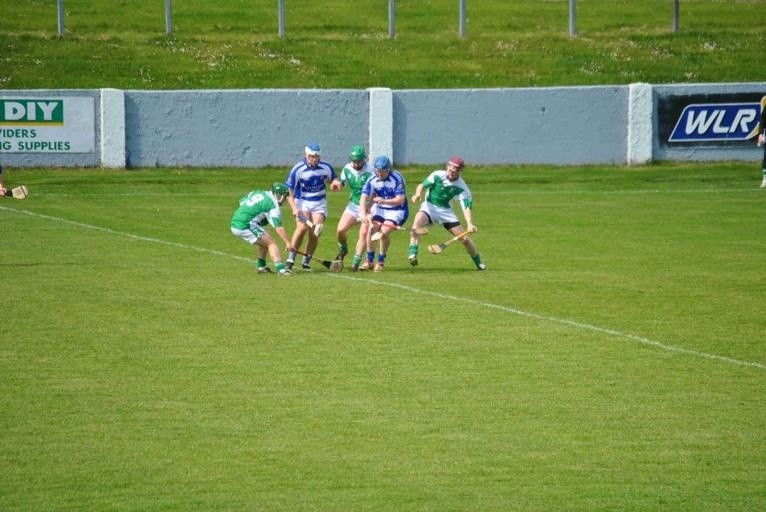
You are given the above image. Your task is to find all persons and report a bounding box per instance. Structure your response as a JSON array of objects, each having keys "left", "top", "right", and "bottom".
[
  {"left": 0, "top": 164, "right": 8, "bottom": 198},
  {"left": 357, "top": 155, "right": 410, "bottom": 272},
  {"left": 229, "top": 181, "right": 296, "bottom": 275},
  {"left": 757, "top": 95, "right": 766, "bottom": 189},
  {"left": 408, "top": 157, "right": 486, "bottom": 270},
  {"left": 285, "top": 143, "right": 343, "bottom": 273},
  {"left": 333, "top": 145, "right": 375, "bottom": 269}
]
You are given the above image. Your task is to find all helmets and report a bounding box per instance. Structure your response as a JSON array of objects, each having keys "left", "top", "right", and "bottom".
[
  {"left": 271, "top": 182, "right": 291, "bottom": 198},
  {"left": 304, "top": 143, "right": 321, "bottom": 164},
  {"left": 350, "top": 144, "right": 366, "bottom": 162},
  {"left": 449, "top": 156, "right": 465, "bottom": 171}
]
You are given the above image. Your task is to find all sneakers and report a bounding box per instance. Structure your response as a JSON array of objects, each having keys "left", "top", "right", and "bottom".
[
  {"left": 373, "top": 253, "right": 386, "bottom": 274},
  {"left": 358, "top": 251, "right": 376, "bottom": 271},
  {"left": 256, "top": 261, "right": 292, "bottom": 276},
  {"left": 477, "top": 262, "right": 486, "bottom": 270},
  {"left": 285, "top": 259, "right": 312, "bottom": 270},
  {"left": 350, "top": 255, "right": 362, "bottom": 271},
  {"left": 408, "top": 254, "right": 418, "bottom": 266},
  {"left": 336, "top": 243, "right": 348, "bottom": 264}
]
